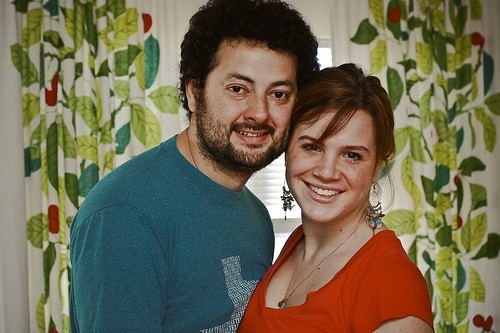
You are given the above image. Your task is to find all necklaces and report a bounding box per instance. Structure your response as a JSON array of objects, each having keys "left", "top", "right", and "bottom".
[
  {"left": 278, "top": 213, "right": 370, "bottom": 310},
  {"left": 185, "top": 131, "right": 198, "bottom": 171}
]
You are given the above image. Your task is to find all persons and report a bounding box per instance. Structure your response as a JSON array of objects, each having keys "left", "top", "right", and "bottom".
[
  {"left": 231, "top": 61, "right": 438, "bottom": 333},
  {"left": 67, "top": 0, "right": 318, "bottom": 333}
]
